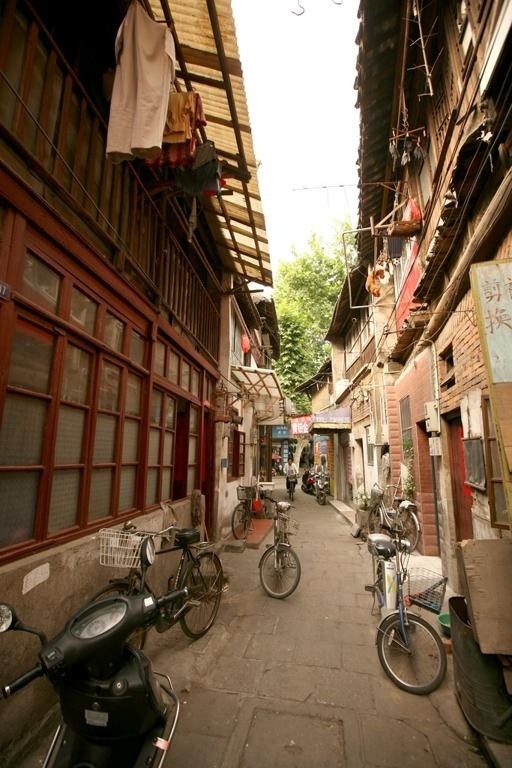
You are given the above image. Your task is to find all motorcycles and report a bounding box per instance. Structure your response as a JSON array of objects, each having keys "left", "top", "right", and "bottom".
[
  {"left": 301, "top": 467, "right": 329, "bottom": 505},
  {"left": 0, "top": 540, "right": 203, "bottom": 768}
]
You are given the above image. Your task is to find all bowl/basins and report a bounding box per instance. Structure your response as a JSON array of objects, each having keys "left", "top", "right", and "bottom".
[{"left": 436, "top": 612, "right": 451, "bottom": 636}]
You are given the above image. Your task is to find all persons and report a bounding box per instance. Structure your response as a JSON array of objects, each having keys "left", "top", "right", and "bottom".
[{"left": 284, "top": 457, "right": 300, "bottom": 493}]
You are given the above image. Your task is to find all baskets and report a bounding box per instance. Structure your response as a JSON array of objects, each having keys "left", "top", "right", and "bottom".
[
  {"left": 278, "top": 512, "right": 299, "bottom": 535},
  {"left": 408, "top": 568, "right": 448, "bottom": 615},
  {"left": 370, "top": 489, "right": 388, "bottom": 505},
  {"left": 237, "top": 486, "right": 255, "bottom": 500},
  {"left": 99, "top": 527, "right": 147, "bottom": 569}
]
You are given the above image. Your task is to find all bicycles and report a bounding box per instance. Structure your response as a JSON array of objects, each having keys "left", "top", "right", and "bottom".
[
  {"left": 287, "top": 474, "right": 297, "bottom": 501},
  {"left": 365, "top": 520, "right": 454, "bottom": 695},
  {"left": 82, "top": 519, "right": 231, "bottom": 651},
  {"left": 230, "top": 485, "right": 258, "bottom": 540},
  {"left": 367, "top": 482, "right": 421, "bottom": 553},
  {"left": 258, "top": 495, "right": 305, "bottom": 600}
]
